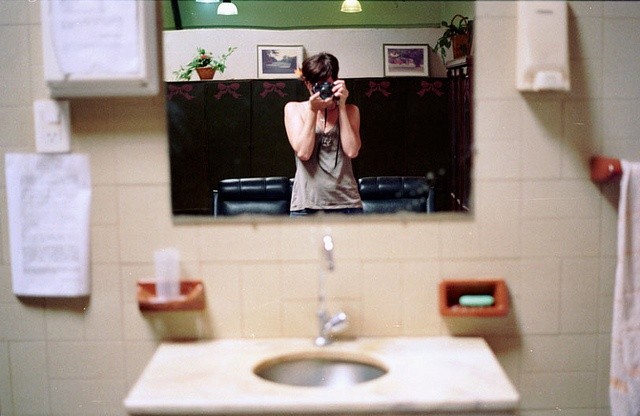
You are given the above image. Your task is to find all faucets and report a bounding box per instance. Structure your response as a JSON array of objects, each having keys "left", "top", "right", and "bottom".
[{"left": 315, "top": 308, "right": 351, "bottom": 347}]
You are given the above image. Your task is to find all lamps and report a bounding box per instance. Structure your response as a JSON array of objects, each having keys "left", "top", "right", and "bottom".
[
  {"left": 215, "top": 0, "right": 245, "bottom": 18},
  {"left": 340, "top": 0, "right": 362, "bottom": 14}
]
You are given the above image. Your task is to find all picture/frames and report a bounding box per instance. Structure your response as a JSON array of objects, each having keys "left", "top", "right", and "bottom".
[
  {"left": 383, "top": 42, "right": 431, "bottom": 79},
  {"left": 258, "top": 44, "right": 304, "bottom": 78}
]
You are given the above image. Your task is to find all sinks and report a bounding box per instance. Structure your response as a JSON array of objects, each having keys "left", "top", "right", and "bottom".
[{"left": 254, "top": 350, "right": 389, "bottom": 387}]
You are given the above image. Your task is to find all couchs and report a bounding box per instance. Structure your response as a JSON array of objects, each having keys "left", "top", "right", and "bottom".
[{"left": 211, "top": 176, "right": 433, "bottom": 217}]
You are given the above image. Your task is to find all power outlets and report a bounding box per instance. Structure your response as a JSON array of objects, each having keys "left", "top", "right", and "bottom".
[{"left": 35, "top": 98, "right": 70, "bottom": 156}]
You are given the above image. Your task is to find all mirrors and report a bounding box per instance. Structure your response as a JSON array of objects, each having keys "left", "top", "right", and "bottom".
[{"left": 163, "top": 0, "right": 474, "bottom": 218}]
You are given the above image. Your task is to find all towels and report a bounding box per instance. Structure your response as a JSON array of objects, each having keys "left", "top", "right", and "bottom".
[
  {"left": 607, "top": 160, "right": 640, "bottom": 416},
  {"left": 5, "top": 153, "right": 93, "bottom": 300}
]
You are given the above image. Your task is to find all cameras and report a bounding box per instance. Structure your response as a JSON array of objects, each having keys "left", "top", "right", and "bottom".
[{"left": 311, "top": 81, "right": 340, "bottom": 103}]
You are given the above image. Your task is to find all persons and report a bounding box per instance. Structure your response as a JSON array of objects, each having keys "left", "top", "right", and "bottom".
[{"left": 284, "top": 52, "right": 364, "bottom": 216}]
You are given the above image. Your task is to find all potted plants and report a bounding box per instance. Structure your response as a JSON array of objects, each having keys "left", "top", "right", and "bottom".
[
  {"left": 174, "top": 48, "right": 239, "bottom": 82},
  {"left": 432, "top": 10, "right": 472, "bottom": 62}
]
